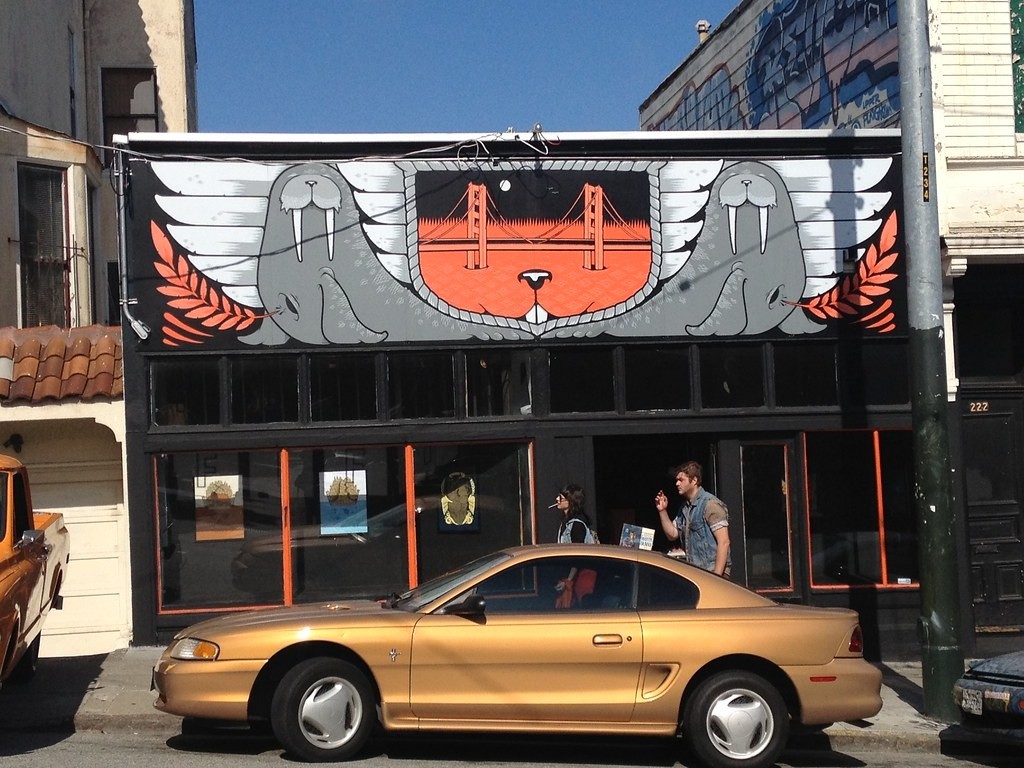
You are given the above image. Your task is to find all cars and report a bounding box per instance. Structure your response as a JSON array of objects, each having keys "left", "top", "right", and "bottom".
[
  {"left": 953, "top": 650, "right": 1024, "bottom": 746},
  {"left": 150, "top": 544, "right": 883, "bottom": 768}
]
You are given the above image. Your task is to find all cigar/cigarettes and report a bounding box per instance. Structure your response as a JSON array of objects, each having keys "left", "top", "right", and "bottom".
[{"left": 548, "top": 503, "right": 558, "bottom": 510}]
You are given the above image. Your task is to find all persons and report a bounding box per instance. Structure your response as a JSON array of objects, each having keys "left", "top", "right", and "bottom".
[
  {"left": 655, "top": 462, "right": 734, "bottom": 583},
  {"left": 547, "top": 481, "right": 603, "bottom": 613}
]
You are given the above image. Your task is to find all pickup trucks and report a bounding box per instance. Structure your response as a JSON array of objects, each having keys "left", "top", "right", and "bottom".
[{"left": 1, "top": 454, "right": 71, "bottom": 692}]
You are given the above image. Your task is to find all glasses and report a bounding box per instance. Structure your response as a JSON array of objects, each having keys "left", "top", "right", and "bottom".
[{"left": 558, "top": 494, "right": 566, "bottom": 503}]
